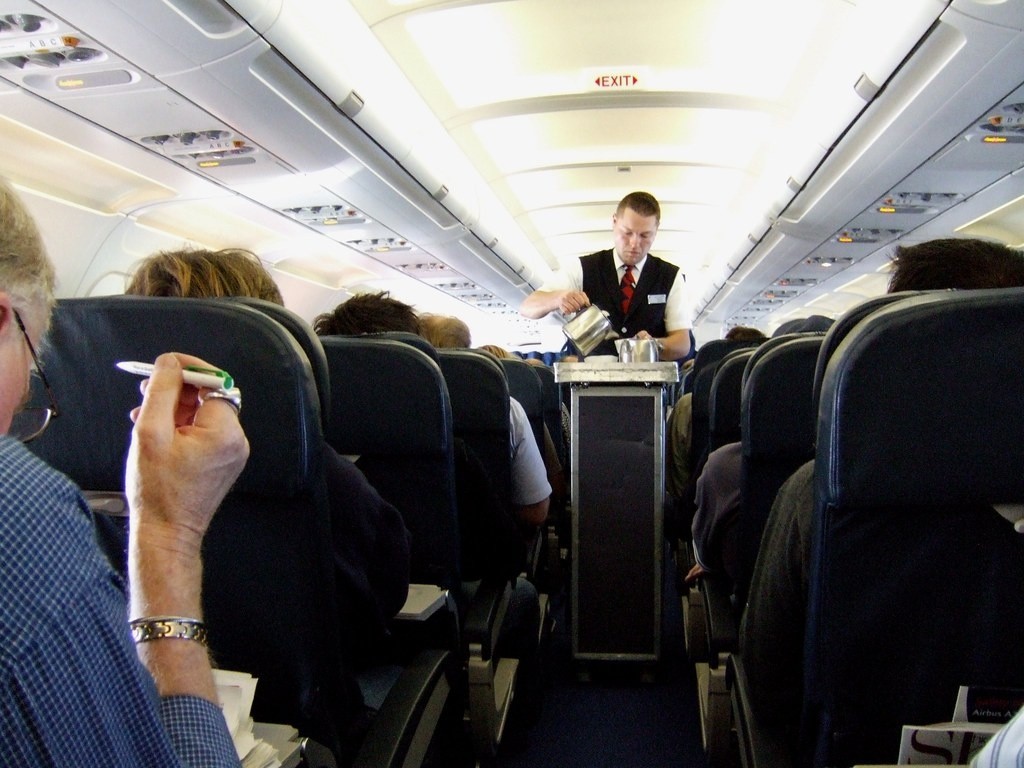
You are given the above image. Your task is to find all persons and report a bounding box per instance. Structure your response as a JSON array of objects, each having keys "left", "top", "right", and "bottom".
[
  {"left": 123, "top": 246, "right": 572, "bottom": 740},
  {"left": 518, "top": 191, "right": 691, "bottom": 377},
  {"left": 666, "top": 238, "right": 1024, "bottom": 768},
  {"left": 0, "top": 175, "right": 250, "bottom": 768}
]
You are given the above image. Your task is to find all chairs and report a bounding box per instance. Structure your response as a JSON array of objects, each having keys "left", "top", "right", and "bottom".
[
  {"left": 7, "top": 296, "right": 562, "bottom": 768},
  {"left": 665, "top": 285, "right": 1023, "bottom": 768}
]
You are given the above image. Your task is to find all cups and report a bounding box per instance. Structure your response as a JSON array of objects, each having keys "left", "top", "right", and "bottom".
[
  {"left": 614, "top": 338, "right": 658, "bottom": 363},
  {"left": 584, "top": 355, "right": 618, "bottom": 362}
]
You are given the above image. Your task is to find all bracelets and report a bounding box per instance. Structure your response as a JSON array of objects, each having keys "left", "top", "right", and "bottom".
[{"left": 128, "top": 615, "right": 208, "bottom": 646}]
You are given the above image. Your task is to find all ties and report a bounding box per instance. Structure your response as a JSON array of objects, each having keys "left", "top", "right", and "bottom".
[{"left": 619, "top": 264, "right": 637, "bottom": 318}]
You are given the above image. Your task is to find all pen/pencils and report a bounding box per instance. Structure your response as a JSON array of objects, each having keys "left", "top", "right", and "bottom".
[{"left": 117, "top": 360, "right": 234, "bottom": 391}]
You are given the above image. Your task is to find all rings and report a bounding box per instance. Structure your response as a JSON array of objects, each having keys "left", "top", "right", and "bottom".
[{"left": 201, "top": 387, "right": 241, "bottom": 415}]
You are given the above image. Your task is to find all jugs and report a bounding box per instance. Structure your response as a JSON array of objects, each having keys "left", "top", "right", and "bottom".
[{"left": 560, "top": 303, "right": 619, "bottom": 357}]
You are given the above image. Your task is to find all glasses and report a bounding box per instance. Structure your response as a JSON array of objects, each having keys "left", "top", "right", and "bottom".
[{"left": 10, "top": 306, "right": 61, "bottom": 442}]
